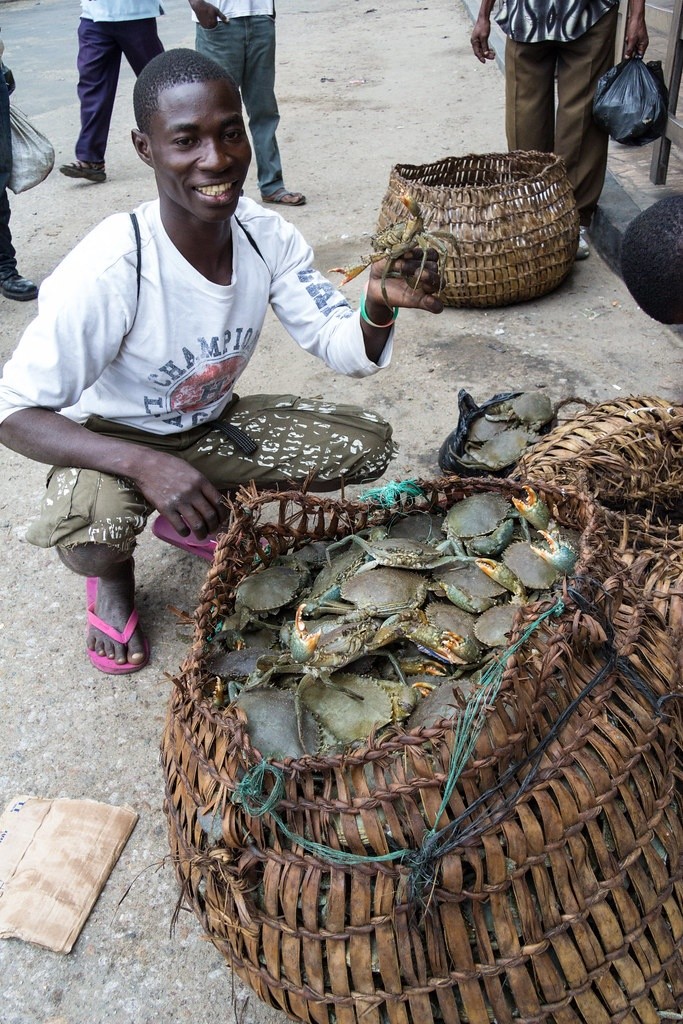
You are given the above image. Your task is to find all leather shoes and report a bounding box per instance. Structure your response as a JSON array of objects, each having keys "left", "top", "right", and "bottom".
[{"left": 0, "top": 271, "right": 38, "bottom": 301}]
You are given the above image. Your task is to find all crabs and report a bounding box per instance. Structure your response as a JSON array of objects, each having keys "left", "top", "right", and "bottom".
[
  {"left": 169, "top": 484, "right": 682, "bottom": 1024},
  {"left": 449, "top": 390, "right": 555, "bottom": 469},
  {"left": 326, "top": 191, "right": 462, "bottom": 312}
]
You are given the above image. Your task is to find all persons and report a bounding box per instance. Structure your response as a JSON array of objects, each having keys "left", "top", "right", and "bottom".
[
  {"left": 185, "top": 1, "right": 304, "bottom": 204},
  {"left": 0, "top": 28, "right": 40, "bottom": 303},
  {"left": 468, "top": 2, "right": 649, "bottom": 261},
  {"left": 56, "top": 1, "right": 164, "bottom": 186},
  {"left": 1, "top": 47, "right": 448, "bottom": 675}
]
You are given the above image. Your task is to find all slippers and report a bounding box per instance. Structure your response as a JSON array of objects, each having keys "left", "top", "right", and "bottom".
[
  {"left": 85, "top": 574, "right": 150, "bottom": 675},
  {"left": 262, "top": 189, "right": 306, "bottom": 206},
  {"left": 152, "top": 513, "right": 269, "bottom": 562}
]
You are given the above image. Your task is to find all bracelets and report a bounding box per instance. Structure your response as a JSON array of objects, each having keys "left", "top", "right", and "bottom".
[{"left": 360, "top": 280, "right": 400, "bottom": 329}]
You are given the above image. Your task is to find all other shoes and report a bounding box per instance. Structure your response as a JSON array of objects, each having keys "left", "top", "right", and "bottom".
[
  {"left": 60, "top": 160, "right": 106, "bottom": 182},
  {"left": 576, "top": 229, "right": 590, "bottom": 259}
]
